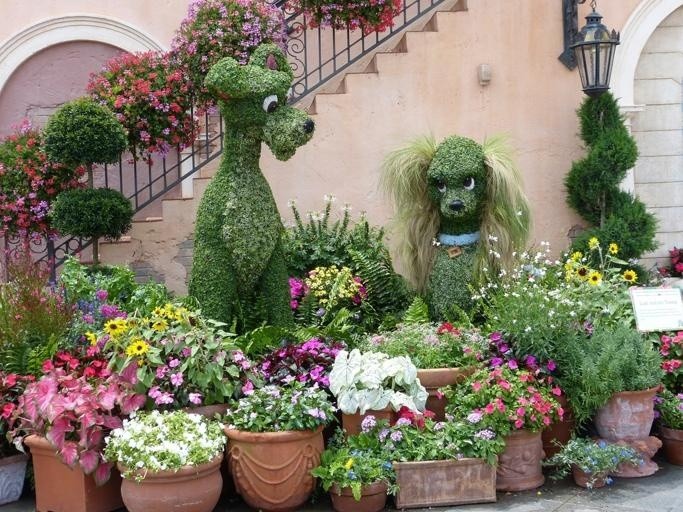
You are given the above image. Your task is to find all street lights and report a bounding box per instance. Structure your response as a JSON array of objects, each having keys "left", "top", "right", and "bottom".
[{"left": 566, "top": 0, "right": 624, "bottom": 234}]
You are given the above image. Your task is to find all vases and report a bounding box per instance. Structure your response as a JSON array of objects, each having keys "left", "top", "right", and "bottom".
[
  {"left": 394, "top": 454, "right": 497, "bottom": 510},
  {"left": 24, "top": 434, "right": 114, "bottom": 509},
  {"left": 600, "top": 385, "right": 662, "bottom": 481},
  {"left": 662, "top": 429, "right": 682, "bottom": 464},
  {"left": 223, "top": 421, "right": 324, "bottom": 509},
  {"left": 342, "top": 407, "right": 392, "bottom": 437},
  {"left": 415, "top": 367, "right": 474, "bottom": 414},
  {"left": 3, "top": 448, "right": 29, "bottom": 503},
  {"left": 328, "top": 484, "right": 388, "bottom": 510},
  {"left": 573, "top": 462, "right": 607, "bottom": 489},
  {"left": 115, "top": 460, "right": 224, "bottom": 509},
  {"left": 496, "top": 427, "right": 546, "bottom": 490}
]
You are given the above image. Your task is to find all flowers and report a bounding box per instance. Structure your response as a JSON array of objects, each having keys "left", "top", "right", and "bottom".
[
  {"left": 449, "top": 361, "right": 565, "bottom": 431},
  {"left": 364, "top": 411, "right": 502, "bottom": 464},
  {"left": 547, "top": 436, "right": 646, "bottom": 472},
  {"left": 5, "top": 355, "right": 134, "bottom": 438},
  {"left": 104, "top": 409, "right": 229, "bottom": 464},
  {"left": 233, "top": 384, "right": 338, "bottom": 424},
  {"left": 660, "top": 391, "right": 682, "bottom": 428},
  {"left": 328, "top": 349, "right": 429, "bottom": 415},
  {"left": 3, "top": 234, "right": 682, "bottom": 385},
  {"left": 315, "top": 428, "right": 397, "bottom": 485},
  {"left": 569, "top": 325, "right": 662, "bottom": 401}
]
